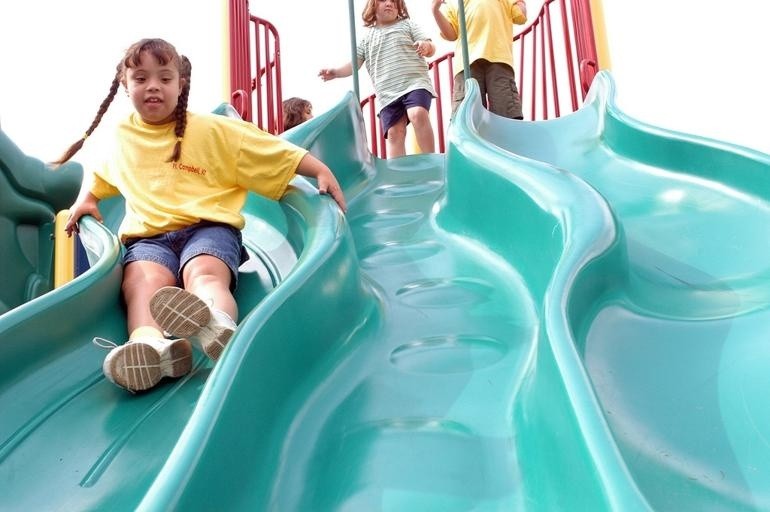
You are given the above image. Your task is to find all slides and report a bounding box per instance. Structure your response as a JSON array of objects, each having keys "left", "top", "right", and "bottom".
[{"left": 0, "top": 70, "right": 769, "bottom": 511}]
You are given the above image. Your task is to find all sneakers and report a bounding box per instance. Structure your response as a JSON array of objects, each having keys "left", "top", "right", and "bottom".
[
  {"left": 149, "top": 286, "right": 235, "bottom": 362},
  {"left": 102, "top": 336, "right": 193, "bottom": 393}
]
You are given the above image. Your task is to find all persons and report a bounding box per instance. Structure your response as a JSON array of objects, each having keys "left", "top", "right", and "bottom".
[
  {"left": 319, "top": 0, "right": 435, "bottom": 158},
  {"left": 275, "top": 97, "right": 314, "bottom": 133},
  {"left": 49, "top": 38, "right": 348, "bottom": 392},
  {"left": 430, "top": 0, "right": 527, "bottom": 121}
]
